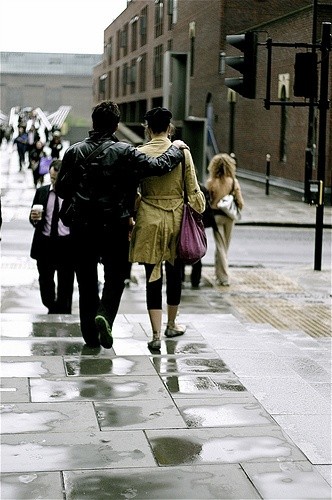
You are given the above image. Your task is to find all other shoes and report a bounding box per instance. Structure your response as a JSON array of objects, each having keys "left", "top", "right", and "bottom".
[
  {"left": 218, "top": 280, "right": 229, "bottom": 285},
  {"left": 148, "top": 340, "right": 161, "bottom": 349},
  {"left": 164, "top": 325, "right": 186, "bottom": 337},
  {"left": 86, "top": 338, "right": 100, "bottom": 349},
  {"left": 95, "top": 315, "right": 113, "bottom": 349}
]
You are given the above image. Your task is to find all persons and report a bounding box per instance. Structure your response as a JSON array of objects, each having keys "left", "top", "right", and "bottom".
[
  {"left": 54, "top": 100, "right": 190, "bottom": 349},
  {"left": 0, "top": 110, "right": 63, "bottom": 188},
  {"left": 29, "top": 160, "right": 74, "bottom": 315},
  {"left": 129, "top": 107, "right": 206, "bottom": 350},
  {"left": 182, "top": 184, "right": 209, "bottom": 290},
  {"left": 206, "top": 153, "right": 244, "bottom": 286}
]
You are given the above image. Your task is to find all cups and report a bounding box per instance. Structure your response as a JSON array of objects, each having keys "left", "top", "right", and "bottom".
[{"left": 33, "top": 205, "right": 43, "bottom": 220}]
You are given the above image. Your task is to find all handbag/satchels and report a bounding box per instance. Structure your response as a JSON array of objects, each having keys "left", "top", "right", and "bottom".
[
  {"left": 39, "top": 157, "right": 51, "bottom": 174},
  {"left": 216, "top": 181, "right": 241, "bottom": 220},
  {"left": 176, "top": 203, "right": 207, "bottom": 265},
  {"left": 59, "top": 194, "right": 75, "bottom": 226}
]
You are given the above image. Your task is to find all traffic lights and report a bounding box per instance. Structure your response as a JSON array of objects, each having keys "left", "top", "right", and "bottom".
[{"left": 224, "top": 30, "right": 257, "bottom": 98}]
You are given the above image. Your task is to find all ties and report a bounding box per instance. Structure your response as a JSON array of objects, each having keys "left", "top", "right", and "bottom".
[{"left": 50, "top": 192, "right": 59, "bottom": 243}]
